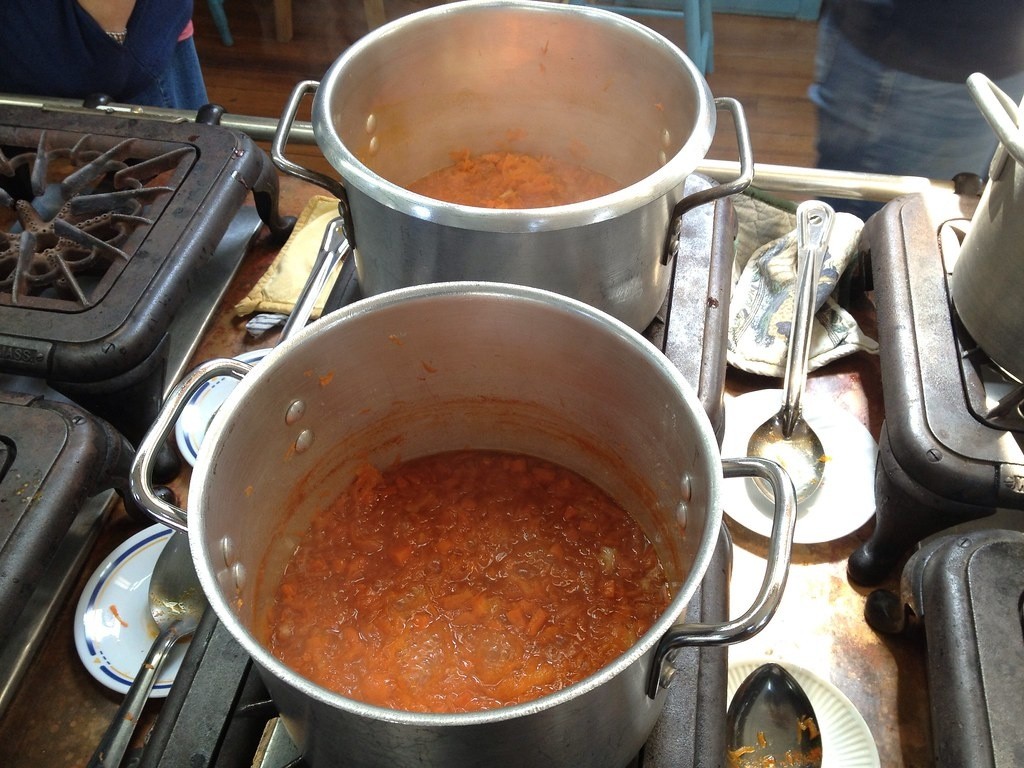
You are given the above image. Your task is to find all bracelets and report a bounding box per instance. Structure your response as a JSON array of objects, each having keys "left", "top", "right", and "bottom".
[{"left": 104, "top": 27, "right": 128, "bottom": 46}]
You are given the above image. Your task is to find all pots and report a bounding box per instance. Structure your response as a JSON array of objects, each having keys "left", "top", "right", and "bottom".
[
  {"left": 268, "top": 1, "right": 756, "bottom": 341},
  {"left": 945, "top": 69, "right": 1024, "bottom": 387}
]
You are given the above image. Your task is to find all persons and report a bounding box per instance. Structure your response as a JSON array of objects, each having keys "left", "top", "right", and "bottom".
[
  {"left": 805, "top": 0, "right": 1024, "bottom": 224},
  {"left": 0, "top": 0, "right": 209, "bottom": 111}
]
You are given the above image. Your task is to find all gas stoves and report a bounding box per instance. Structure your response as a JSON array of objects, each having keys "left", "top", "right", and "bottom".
[{"left": 0, "top": 92, "right": 1024, "bottom": 767}]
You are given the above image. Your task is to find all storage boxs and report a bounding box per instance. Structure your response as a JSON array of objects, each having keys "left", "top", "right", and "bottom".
[
  {"left": 270, "top": 1, "right": 754, "bottom": 333},
  {"left": 132, "top": 281, "right": 797, "bottom": 767}
]
